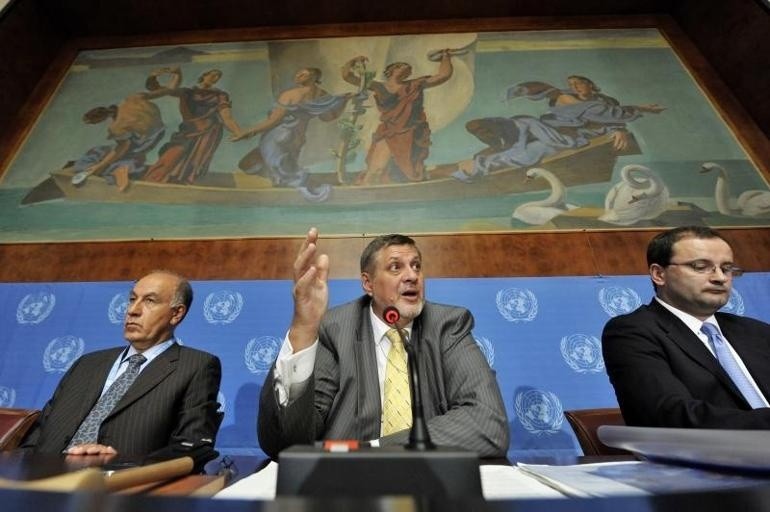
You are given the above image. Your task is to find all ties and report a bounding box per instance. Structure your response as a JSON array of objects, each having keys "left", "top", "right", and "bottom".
[
  {"left": 65, "top": 353, "right": 147, "bottom": 450},
  {"left": 380, "top": 328, "right": 414, "bottom": 436},
  {"left": 701, "top": 323, "right": 768, "bottom": 411}
]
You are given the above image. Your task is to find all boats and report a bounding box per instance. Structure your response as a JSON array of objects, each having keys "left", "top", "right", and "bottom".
[{"left": 52, "top": 132, "right": 635, "bottom": 200}]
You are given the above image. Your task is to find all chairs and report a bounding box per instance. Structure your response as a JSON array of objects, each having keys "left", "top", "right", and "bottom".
[
  {"left": 0, "top": 408, "right": 41, "bottom": 451},
  {"left": 212, "top": 402, "right": 224, "bottom": 450},
  {"left": 562, "top": 407, "right": 630, "bottom": 454}
]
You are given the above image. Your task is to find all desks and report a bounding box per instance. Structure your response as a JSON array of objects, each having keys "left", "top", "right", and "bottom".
[{"left": 0, "top": 447, "right": 769, "bottom": 511}]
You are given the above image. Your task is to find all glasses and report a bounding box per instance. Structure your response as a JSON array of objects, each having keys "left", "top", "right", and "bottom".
[{"left": 661, "top": 258, "right": 743, "bottom": 278}]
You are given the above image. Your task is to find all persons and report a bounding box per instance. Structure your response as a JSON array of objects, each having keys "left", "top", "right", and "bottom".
[
  {"left": 229, "top": 69, "right": 350, "bottom": 189},
  {"left": 0, "top": 271, "right": 223, "bottom": 467},
  {"left": 463, "top": 76, "right": 667, "bottom": 175},
  {"left": 342, "top": 50, "right": 454, "bottom": 181},
  {"left": 602, "top": 225, "right": 770, "bottom": 427},
  {"left": 256, "top": 226, "right": 510, "bottom": 464},
  {"left": 71, "top": 63, "right": 181, "bottom": 183},
  {"left": 148, "top": 67, "right": 243, "bottom": 189}
]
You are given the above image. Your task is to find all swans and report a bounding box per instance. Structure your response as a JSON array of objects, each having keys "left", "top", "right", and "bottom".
[
  {"left": 511, "top": 166, "right": 583, "bottom": 226},
  {"left": 698, "top": 161, "right": 769, "bottom": 219},
  {"left": 595, "top": 164, "right": 670, "bottom": 226}
]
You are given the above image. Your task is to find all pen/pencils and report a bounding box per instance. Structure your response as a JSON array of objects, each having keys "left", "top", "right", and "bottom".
[{"left": 323, "top": 440, "right": 370, "bottom": 451}]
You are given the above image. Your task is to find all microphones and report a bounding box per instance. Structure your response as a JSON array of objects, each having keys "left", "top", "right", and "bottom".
[{"left": 382, "top": 305, "right": 443, "bottom": 451}]
